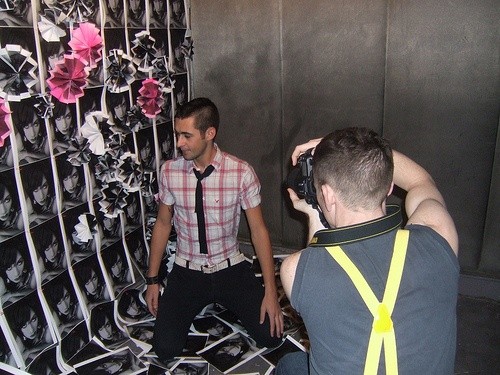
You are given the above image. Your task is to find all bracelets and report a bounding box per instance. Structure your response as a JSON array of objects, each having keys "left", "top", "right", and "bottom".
[{"left": 145, "top": 277, "right": 158, "bottom": 285}]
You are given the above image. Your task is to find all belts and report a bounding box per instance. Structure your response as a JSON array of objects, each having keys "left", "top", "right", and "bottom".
[{"left": 174, "top": 253, "right": 245, "bottom": 274}]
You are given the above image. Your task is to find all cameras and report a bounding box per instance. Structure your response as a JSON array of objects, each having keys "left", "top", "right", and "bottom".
[{"left": 283, "top": 147, "right": 325, "bottom": 223}]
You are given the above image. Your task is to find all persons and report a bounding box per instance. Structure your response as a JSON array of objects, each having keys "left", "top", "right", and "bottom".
[
  {"left": 274, "top": 127, "right": 462, "bottom": 375},
  {"left": 0, "top": 0, "right": 310, "bottom": 375},
  {"left": 144, "top": 97, "right": 284, "bottom": 360}
]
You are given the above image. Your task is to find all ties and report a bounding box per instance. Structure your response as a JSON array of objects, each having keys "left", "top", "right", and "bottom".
[{"left": 193, "top": 164, "right": 216, "bottom": 255}]
